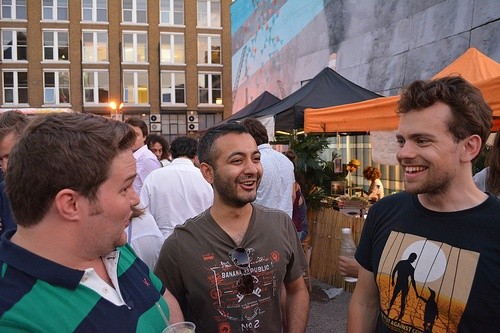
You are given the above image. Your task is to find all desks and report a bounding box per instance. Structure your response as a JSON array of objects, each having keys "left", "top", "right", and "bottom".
[{"left": 335, "top": 206, "right": 365, "bottom": 218}]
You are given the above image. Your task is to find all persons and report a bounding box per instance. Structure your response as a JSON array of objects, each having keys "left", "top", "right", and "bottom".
[
  {"left": 0, "top": 113, "right": 186, "bottom": 333},
  {"left": 240, "top": 119, "right": 296, "bottom": 220},
  {"left": 139, "top": 137, "right": 214, "bottom": 240},
  {"left": 124, "top": 117, "right": 163, "bottom": 197},
  {"left": 0, "top": 110, "right": 34, "bottom": 232},
  {"left": 144, "top": 134, "right": 168, "bottom": 161},
  {"left": 281, "top": 151, "right": 307, "bottom": 240},
  {"left": 153, "top": 124, "right": 310, "bottom": 333},
  {"left": 363, "top": 166, "right": 384, "bottom": 201},
  {"left": 337, "top": 159, "right": 360, "bottom": 178},
  {"left": 346, "top": 76, "right": 500, "bottom": 333}
]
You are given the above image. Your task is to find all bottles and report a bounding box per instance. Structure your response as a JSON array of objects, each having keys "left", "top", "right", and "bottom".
[{"left": 340, "top": 228, "right": 357, "bottom": 282}]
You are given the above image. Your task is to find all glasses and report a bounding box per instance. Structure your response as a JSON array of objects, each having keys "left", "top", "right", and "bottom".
[{"left": 228, "top": 245, "right": 254, "bottom": 294}]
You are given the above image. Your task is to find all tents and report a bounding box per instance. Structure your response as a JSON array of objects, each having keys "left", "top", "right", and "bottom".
[
  {"left": 227, "top": 66, "right": 386, "bottom": 137},
  {"left": 304, "top": 47, "right": 500, "bottom": 194},
  {"left": 217, "top": 91, "right": 282, "bottom": 124}
]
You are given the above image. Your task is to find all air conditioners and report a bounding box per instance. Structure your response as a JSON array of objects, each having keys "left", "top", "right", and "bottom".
[
  {"left": 188, "top": 114, "right": 198, "bottom": 131},
  {"left": 150, "top": 114, "right": 162, "bottom": 131}
]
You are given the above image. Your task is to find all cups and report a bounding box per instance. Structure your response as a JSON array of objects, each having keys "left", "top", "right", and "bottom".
[{"left": 162, "top": 322, "right": 196, "bottom": 333}]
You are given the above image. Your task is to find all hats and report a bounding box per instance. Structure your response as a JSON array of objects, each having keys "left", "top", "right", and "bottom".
[{"left": 349, "top": 159, "right": 360, "bottom": 169}]
